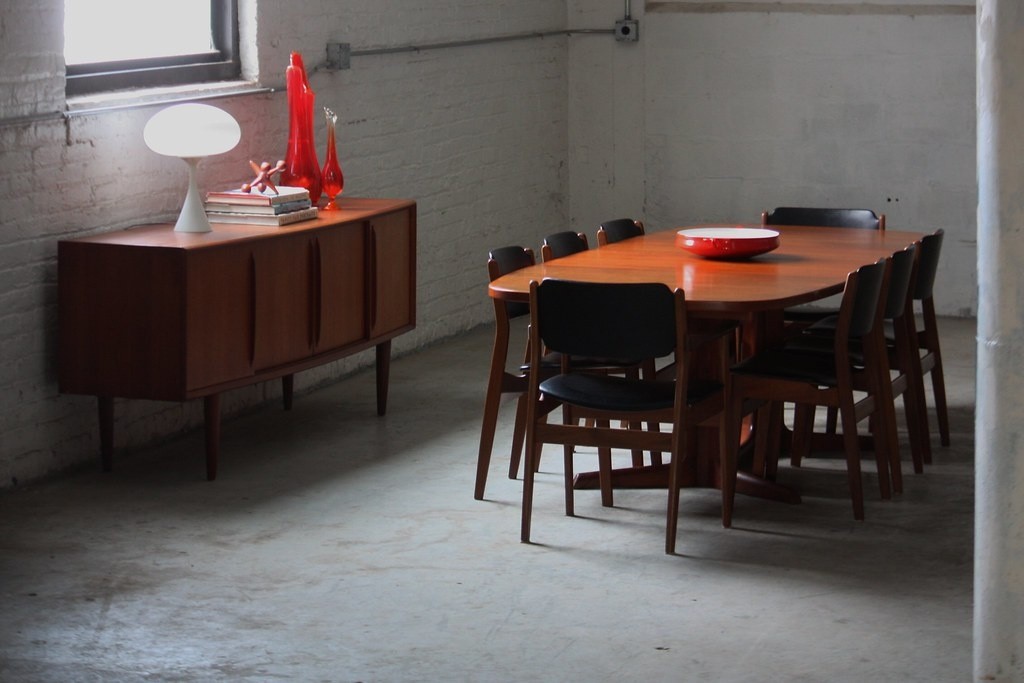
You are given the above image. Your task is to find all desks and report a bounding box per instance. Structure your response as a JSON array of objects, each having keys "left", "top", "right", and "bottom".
[{"left": 485, "top": 224, "right": 926, "bottom": 503}]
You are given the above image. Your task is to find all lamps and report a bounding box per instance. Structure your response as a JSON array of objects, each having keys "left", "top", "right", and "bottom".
[{"left": 144, "top": 102, "right": 242, "bottom": 233}]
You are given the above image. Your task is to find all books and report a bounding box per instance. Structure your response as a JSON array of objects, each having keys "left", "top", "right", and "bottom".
[{"left": 205, "top": 185, "right": 318, "bottom": 226}]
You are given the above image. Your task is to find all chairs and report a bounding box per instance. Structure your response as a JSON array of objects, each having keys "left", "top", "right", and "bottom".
[
  {"left": 533, "top": 231, "right": 663, "bottom": 473},
  {"left": 730, "top": 257, "right": 898, "bottom": 519},
  {"left": 520, "top": 275, "right": 731, "bottom": 556},
  {"left": 474, "top": 243, "right": 613, "bottom": 508},
  {"left": 803, "top": 226, "right": 951, "bottom": 468},
  {"left": 597, "top": 218, "right": 641, "bottom": 243},
  {"left": 762, "top": 205, "right": 886, "bottom": 329},
  {"left": 766, "top": 239, "right": 925, "bottom": 501}
]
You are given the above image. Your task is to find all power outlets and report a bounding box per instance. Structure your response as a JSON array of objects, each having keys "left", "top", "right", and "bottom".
[
  {"left": 614, "top": 19, "right": 639, "bottom": 42},
  {"left": 327, "top": 42, "right": 350, "bottom": 69}
]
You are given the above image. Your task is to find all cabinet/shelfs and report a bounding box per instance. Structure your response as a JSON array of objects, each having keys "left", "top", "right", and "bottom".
[{"left": 51, "top": 198, "right": 417, "bottom": 480}]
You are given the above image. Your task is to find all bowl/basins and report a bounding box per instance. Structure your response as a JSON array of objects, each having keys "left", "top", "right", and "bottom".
[{"left": 677, "top": 228, "right": 780, "bottom": 258}]
